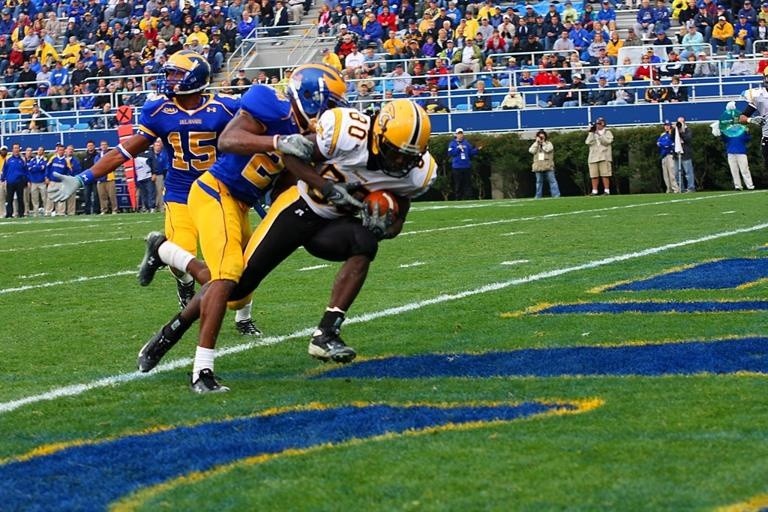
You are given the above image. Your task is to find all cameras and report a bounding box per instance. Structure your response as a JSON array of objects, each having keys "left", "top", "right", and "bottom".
[
  {"left": 589, "top": 122, "right": 597, "bottom": 131},
  {"left": 676, "top": 122, "right": 682, "bottom": 128}
]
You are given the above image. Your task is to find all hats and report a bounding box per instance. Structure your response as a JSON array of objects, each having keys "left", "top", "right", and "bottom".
[
  {"left": 0, "top": 0, "right": 222, "bottom": 45},
  {"left": 23, "top": 48, "right": 144, "bottom": 97},
  {"left": 338, "top": 6, "right": 353, "bottom": 31},
  {"left": 356, "top": 0, "right": 768, "bottom": 88}
]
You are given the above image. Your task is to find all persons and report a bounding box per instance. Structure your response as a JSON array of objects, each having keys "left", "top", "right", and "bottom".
[
  {"left": 0, "top": 0, "right": 767, "bottom": 219},
  {"left": 46, "top": 49, "right": 263, "bottom": 339},
  {"left": 136, "top": 62, "right": 350, "bottom": 395},
  {"left": 137, "top": 97, "right": 440, "bottom": 362}
]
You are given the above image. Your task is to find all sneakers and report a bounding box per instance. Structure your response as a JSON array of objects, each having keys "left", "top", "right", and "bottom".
[
  {"left": 174, "top": 275, "right": 196, "bottom": 312},
  {"left": 136, "top": 230, "right": 169, "bottom": 288},
  {"left": 135, "top": 320, "right": 176, "bottom": 374},
  {"left": 4, "top": 204, "right": 156, "bottom": 220},
  {"left": 187, "top": 366, "right": 232, "bottom": 397},
  {"left": 235, "top": 318, "right": 264, "bottom": 339},
  {"left": 307, "top": 325, "right": 358, "bottom": 364}
]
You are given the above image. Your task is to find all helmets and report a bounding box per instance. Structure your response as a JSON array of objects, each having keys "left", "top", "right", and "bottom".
[
  {"left": 155, "top": 49, "right": 211, "bottom": 100},
  {"left": 366, "top": 95, "right": 433, "bottom": 180},
  {"left": 285, "top": 62, "right": 349, "bottom": 130}
]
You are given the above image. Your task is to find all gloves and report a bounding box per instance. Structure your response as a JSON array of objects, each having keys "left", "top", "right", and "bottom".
[
  {"left": 359, "top": 200, "right": 401, "bottom": 242},
  {"left": 46, "top": 170, "right": 82, "bottom": 203},
  {"left": 323, "top": 176, "right": 367, "bottom": 218},
  {"left": 272, "top": 133, "right": 315, "bottom": 162}
]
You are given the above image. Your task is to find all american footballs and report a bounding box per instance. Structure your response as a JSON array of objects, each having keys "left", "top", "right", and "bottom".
[{"left": 364, "top": 190, "right": 400, "bottom": 221}]
